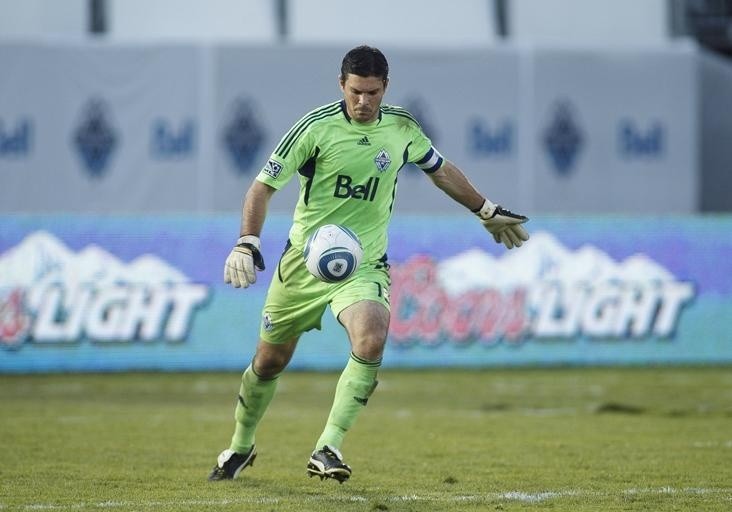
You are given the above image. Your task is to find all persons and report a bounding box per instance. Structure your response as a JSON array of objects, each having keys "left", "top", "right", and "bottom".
[{"left": 207, "top": 43, "right": 531, "bottom": 483}]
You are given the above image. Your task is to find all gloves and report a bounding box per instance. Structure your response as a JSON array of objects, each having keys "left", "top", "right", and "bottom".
[
  {"left": 224, "top": 234, "right": 266, "bottom": 290},
  {"left": 471, "top": 198, "right": 532, "bottom": 250}
]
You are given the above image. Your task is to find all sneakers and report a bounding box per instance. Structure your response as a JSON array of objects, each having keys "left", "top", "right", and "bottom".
[
  {"left": 206, "top": 442, "right": 258, "bottom": 482},
  {"left": 305, "top": 444, "right": 352, "bottom": 483}
]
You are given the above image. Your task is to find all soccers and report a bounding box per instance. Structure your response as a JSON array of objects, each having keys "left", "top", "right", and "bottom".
[{"left": 303, "top": 224, "right": 364, "bottom": 283}]
